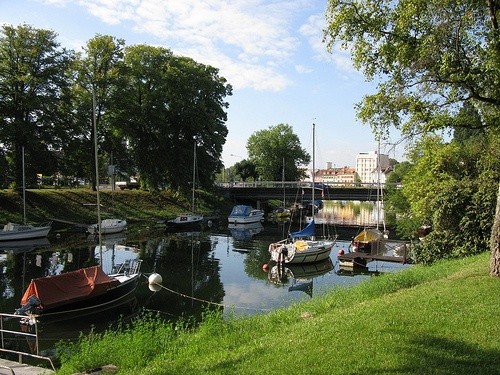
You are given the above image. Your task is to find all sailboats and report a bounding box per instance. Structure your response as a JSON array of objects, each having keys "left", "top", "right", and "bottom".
[
  {"left": 350, "top": 134, "right": 388, "bottom": 249},
  {"left": 0, "top": 148, "right": 52, "bottom": 240},
  {"left": 170, "top": 142, "right": 205, "bottom": 225},
  {"left": 268, "top": 117, "right": 338, "bottom": 265}
]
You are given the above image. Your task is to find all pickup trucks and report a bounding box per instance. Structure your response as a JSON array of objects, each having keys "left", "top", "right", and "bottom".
[{"left": 116, "top": 176, "right": 141, "bottom": 190}]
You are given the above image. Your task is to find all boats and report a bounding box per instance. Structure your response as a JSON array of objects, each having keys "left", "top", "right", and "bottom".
[
  {"left": 228, "top": 222, "right": 265, "bottom": 239},
  {"left": 269, "top": 209, "right": 292, "bottom": 218},
  {"left": 0, "top": 265, "right": 143, "bottom": 326},
  {"left": 228, "top": 206, "right": 264, "bottom": 224},
  {"left": 289, "top": 201, "right": 320, "bottom": 211},
  {"left": 87, "top": 218, "right": 127, "bottom": 235},
  {"left": 264, "top": 256, "right": 334, "bottom": 298}
]
